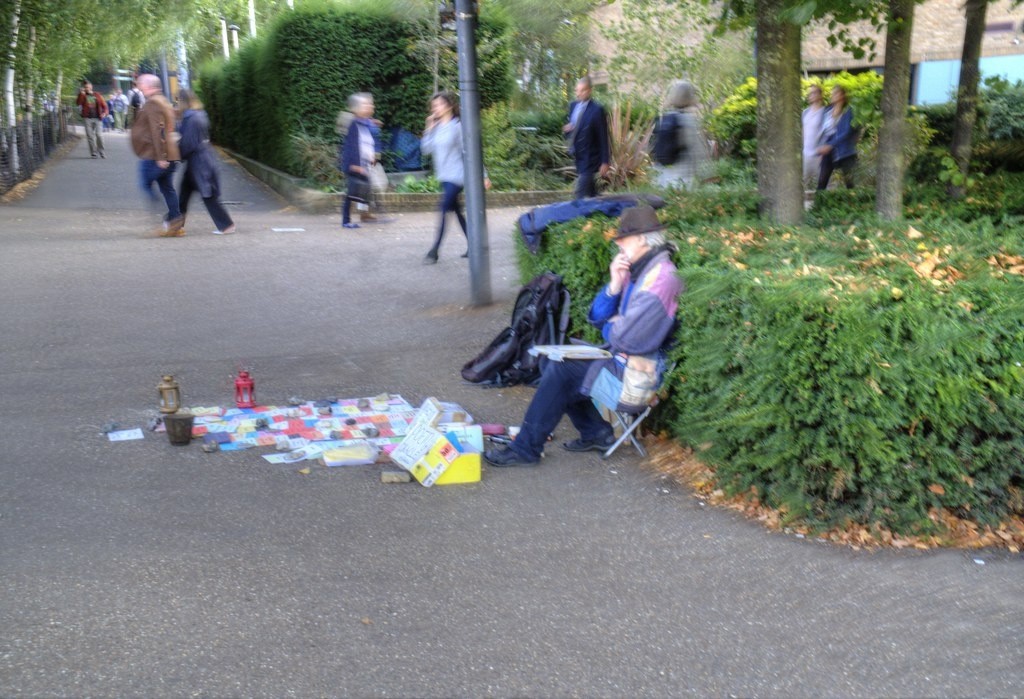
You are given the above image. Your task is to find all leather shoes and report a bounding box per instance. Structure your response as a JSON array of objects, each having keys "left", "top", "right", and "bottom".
[{"left": 211, "top": 227, "right": 236, "bottom": 234}]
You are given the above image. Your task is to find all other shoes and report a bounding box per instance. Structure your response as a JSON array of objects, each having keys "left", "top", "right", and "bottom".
[
  {"left": 100, "top": 153, "right": 108, "bottom": 159},
  {"left": 421, "top": 254, "right": 439, "bottom": 265},
  {"left": 360, "top": 213, "right": 378, "bottom": 222},
  {"left": 342, "top": 221, "right": 358, "bottom": 229},
  {"left": 161, "top": 222, "right": 186, "bottom": 237},
  {"left": 91, "top": 153, "right": 99, "bottom": 159},
  {"left": 461, "top": 249, "right": 469, "bottom": 257}
]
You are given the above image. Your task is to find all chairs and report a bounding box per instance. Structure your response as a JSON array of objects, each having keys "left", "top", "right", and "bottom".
[{"left": 602, "top": 362, "right": 677, "bottom": 460}]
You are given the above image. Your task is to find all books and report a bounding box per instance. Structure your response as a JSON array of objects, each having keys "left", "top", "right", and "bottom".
[{"left": 323, "top": 446, "right": 374, "bottom": 467}]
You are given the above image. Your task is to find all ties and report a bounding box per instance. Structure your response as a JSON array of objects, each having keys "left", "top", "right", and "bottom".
[{"left": 562, "top": 106, "right": 585, "bottom": 152}]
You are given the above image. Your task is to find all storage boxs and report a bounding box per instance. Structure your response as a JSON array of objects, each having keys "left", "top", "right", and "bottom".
[{"left": 387, "top": 398, "right": 484, "bottom": 487}]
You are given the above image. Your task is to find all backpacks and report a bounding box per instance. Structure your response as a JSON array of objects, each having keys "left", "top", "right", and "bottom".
[
  {"left": 130, "top": 89, "right": 141, "bottom": 109},
  {"left": 652, "top": 113, "right": 688, "bottom": 166}
]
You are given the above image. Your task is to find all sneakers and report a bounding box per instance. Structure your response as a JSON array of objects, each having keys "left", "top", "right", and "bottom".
[
  {"left": 482, "top": 447, "right": 540, "bottom": 467},
  {"left": 562, "top": 428, "right": 616, "bottom": 452}
]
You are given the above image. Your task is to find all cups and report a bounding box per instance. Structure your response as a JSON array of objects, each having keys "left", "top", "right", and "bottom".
[{"left": 163, "top": 414, "right": 193, "bottom": 445}]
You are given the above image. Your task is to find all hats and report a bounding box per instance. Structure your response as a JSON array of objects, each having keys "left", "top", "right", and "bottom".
[
  {"left": 601, "top": 204, "right": 672, "bottom": 239},
  {"left": 663, "top": 79, "right": 694, "bottom": 107}
]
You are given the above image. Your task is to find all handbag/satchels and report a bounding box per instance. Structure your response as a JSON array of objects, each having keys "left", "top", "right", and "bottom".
[
  {"left": 346, "top": 172, "right": 371, "bottom": 204},
  {"left": 462, "top": 268, "right": 573, "bottom": 387}
]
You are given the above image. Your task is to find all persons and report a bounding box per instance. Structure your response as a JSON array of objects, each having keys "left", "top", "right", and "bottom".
[
  {"left": 102, "top": 80, "right": 145, "bottom": 132},
  {"left": 649, "top": 81, "right": 722, "bottom": 186},
  {"left": 163, "top": 89, "right": 234, "bottom": 234},
  {"left": 561, "top": 77, "right": 610, "bottom": 199},
  {"left": 132, "top": 74, "right": 181, "bottom": 221},
  {"left": 337, "top": 93, "right": 389, "bottom": 229},
  {"left": 482, "top": 205, "right": 688, "bottom": 466},
  {"left": 818, "top": 84, "right": 857, "bottom": 189},
  {"left": 421, "top": 92, "right": 491, "bottom": 264},
  {"left": 77, "top": 80, "right": 108, "bottom": 159},
  {"left": 800, "top": 84, "right": 826, "bottom": 189}
]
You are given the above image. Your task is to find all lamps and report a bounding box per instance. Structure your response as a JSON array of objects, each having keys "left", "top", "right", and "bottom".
[
  {"left": 157, "top": 376, "right": 181, "bottom": 414},
  {"left": 235, "top": 372, "right": 256, "bottom": 407}
]
